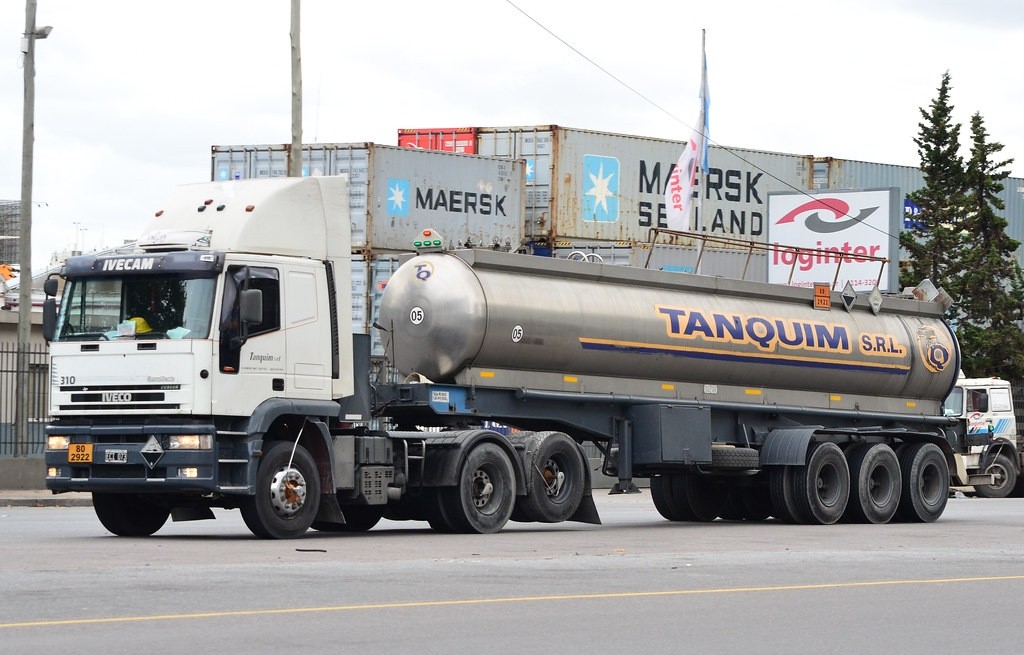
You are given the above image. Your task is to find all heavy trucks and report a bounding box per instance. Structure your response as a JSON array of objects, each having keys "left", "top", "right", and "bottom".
[
  {"left": 946, "top": 369, "right": 1024, "bottom": 499},
  {"left": 40, "top": 172, "right": 964, "bottom": 541}
]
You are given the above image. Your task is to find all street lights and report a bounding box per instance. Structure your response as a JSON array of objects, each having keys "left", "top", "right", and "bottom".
[{"left": 13, "top": 22, "right": 52, "bottom": 457}]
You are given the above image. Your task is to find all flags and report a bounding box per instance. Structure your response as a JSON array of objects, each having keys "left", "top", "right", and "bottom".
[{"left": 662, "top": 105, "right": 707, "bottom": 233}]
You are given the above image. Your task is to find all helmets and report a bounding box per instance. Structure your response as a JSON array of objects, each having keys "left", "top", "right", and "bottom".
[{"left": 130, "top": 317, "right": 152, "bottom": 334}]
